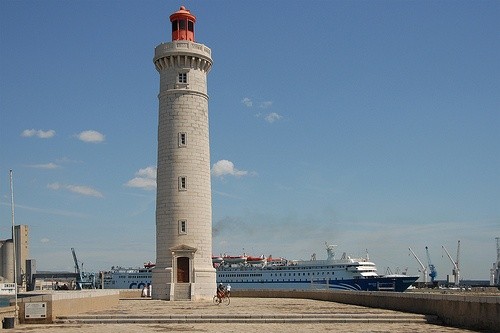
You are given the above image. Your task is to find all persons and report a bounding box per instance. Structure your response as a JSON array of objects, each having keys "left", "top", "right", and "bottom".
[
  {"left": 142, "top": 286, "right": 148, "bottom": 296},
  {"left": 227, "top": 283, "right": 231, "bottom": 297},
  {"left": 217, "top": 283, "right": 225, "bottom": 303},
  {"left": 148, "top": 284, "right": 152, "bottom": 297}
]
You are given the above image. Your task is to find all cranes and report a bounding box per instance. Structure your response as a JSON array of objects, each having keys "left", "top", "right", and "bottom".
[
  {"left": 71, "top": 247, "right": 95, "bottom": 289},
  {"left": 409, "top": 235, "right": 500, "bottom": 287}
]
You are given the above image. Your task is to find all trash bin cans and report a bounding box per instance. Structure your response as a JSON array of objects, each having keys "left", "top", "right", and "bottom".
[{"left": 2, "top": 317, "right": 15, "bottom": 329}]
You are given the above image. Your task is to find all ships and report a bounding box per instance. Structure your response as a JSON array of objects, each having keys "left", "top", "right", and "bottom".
[{"left": 100, "top": 238, "right": 421, "bottom": 293}]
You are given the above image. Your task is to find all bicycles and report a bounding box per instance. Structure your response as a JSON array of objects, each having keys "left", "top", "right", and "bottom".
[{"left": 212, "top": 290, "right": 230, "bottom": 307}]
